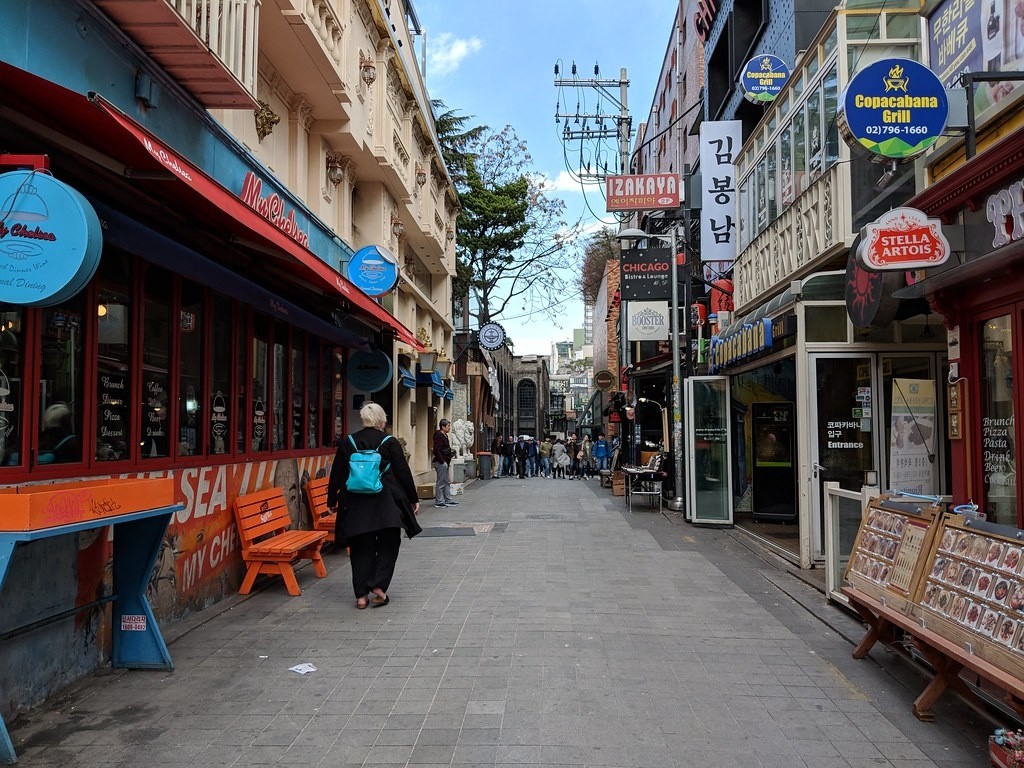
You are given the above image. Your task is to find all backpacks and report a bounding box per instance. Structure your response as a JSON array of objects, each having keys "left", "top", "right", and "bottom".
[{"left": 345, "top": 434, "right": 392, "bottom": 493}]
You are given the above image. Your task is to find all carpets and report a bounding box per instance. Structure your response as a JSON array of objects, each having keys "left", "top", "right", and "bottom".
[{"left": 404, "top": 527, "right": 476, "bottom": 538}]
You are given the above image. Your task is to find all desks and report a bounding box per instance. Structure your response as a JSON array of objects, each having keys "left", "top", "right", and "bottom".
[
  {"left": 0, "top": 505, "right": 186, "bottom": 764},
  {"left": 621, "top": 466, "right": 667, "bottom": 515}
]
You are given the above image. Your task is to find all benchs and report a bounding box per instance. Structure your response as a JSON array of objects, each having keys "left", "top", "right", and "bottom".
[
  {"left": 841, "top": 586, "right": 1024, "bottom": 723},
  {"left": 305, "top": 476, "right": 350, "bottom": 556},
  {"left": 233, "top": 487, "right": 328, "bottom": 596}
]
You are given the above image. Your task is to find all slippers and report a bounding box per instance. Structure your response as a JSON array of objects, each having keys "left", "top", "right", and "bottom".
[
  {"left": 371, "top": 594, "right": 390, "bottom": 604},
  {"left": 356, "top": 599, "right": 369, "bottom": 609}
]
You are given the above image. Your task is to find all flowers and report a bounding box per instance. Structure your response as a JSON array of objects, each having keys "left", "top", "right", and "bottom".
[{"left": 994, "top": 728, "right": 1024, "bottom": 768}]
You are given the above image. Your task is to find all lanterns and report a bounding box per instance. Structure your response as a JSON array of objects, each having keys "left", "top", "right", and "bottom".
[
  {"left": 710, "top": 278, "right": 733, "bottom": 319},
  {"left": 691, "top": 303, "right": 707, "bottom": 329}
]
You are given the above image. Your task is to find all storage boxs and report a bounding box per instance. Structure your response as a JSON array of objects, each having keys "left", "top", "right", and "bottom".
[
  {"left": 449, "top": 482, "right": 464, "bottom": 495},
  {"left": 613, "top": 472, "right": 626, "bottom": 495},
  {"left": 417, "top": 483, "right": 436, "bottom": 498},
  {"left": 0, "top": 478, "right": 174, "bottom": 531}
]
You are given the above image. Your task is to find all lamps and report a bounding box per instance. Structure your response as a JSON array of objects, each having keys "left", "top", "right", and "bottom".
[
  {"left": 446, "top": 225, "right": 454, "bottom": 243},
  {"left": 405, "top": 255, "right": 416, "bottom": 281},
  {"left": 638, "top": 398, "right": 665, "bottom": 411},
  {"left": 390, "top": 211, "right": 404, "bottom": 239},
  {"left": 415, "top": 346, "right": 451, "bottom": 379},
  {"left": 920, "top": 314, "right": 935, "bottom": 338},
  {"left": 415, "top": 161, "right": 426, "bottom": 189},
  {"left": 326, "top": 155, "right": 345, "bottom": 192},
  {"left": 398, "top": 333, "right": 438, "bottom": 373},
  {"left": 360, "top": 50, "right": 376, "bottom": 90}
]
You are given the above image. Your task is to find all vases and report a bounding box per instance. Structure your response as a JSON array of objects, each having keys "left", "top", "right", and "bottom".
[{"left": 989, "top": 736, "right": 1015, "bottom": 768}]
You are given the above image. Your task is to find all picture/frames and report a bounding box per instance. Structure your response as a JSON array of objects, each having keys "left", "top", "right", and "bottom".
[
  {"left": 947, "top": 383, "right": 961, "bottom": 411},
  {"left": 948, "top": 411, "right": 962, "bottom": 440}
]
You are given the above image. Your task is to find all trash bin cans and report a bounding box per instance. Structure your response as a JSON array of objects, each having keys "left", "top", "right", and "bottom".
[{"left": 476, "top": 451, "right": 493, "bottom": 480}]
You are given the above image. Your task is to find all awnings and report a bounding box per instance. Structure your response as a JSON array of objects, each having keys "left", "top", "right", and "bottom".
[
  {"left": 444, "top": 386, "right": 455, "bottom": 400},
  {"left": 88, "top": 94, "right": 426, "bottom": 358},
  {"left": 416, "top": 368, "right": 445, "bottom": 398},
  {"left": 398, "top": 360, "right": 416, "bottom": 390},
  {"left": 620, "top": 349, "right": 685, "bottom": 379}
]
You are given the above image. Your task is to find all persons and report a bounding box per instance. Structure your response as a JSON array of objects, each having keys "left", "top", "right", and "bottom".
[
  {"left": 431, "top": 419, "right": 460, "bottom": 508},
  {"left": 330, "top": 403, "right": 423, "bottom": 609},
  {"left": 592, "top": 433, "right": 612, "bottom": 481},
  {"left": 503, "top": 435, "right": 571, "bottom": 479},
  {"left": 566, "top": 432, "right": 582, "bottom": 480},
  {"left": 580, "top": 434, "right": 595, "bottom": 481},
  {"left": 490, "top": 432, "right": 502, "bottom": 479},
  {"left": 497, "top": 435, "right": 504, "bottom": 479}
]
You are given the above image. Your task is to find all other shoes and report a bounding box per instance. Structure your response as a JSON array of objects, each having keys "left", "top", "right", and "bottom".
[
  {"left": 445, "top": 502, "right": 459, "bottom": 506},
  {"left": 516, "top": 474, "right": 601, "bottom": 481},
  {"left": 435, "top": 503, "right": 448, "bottom": 508}
]
